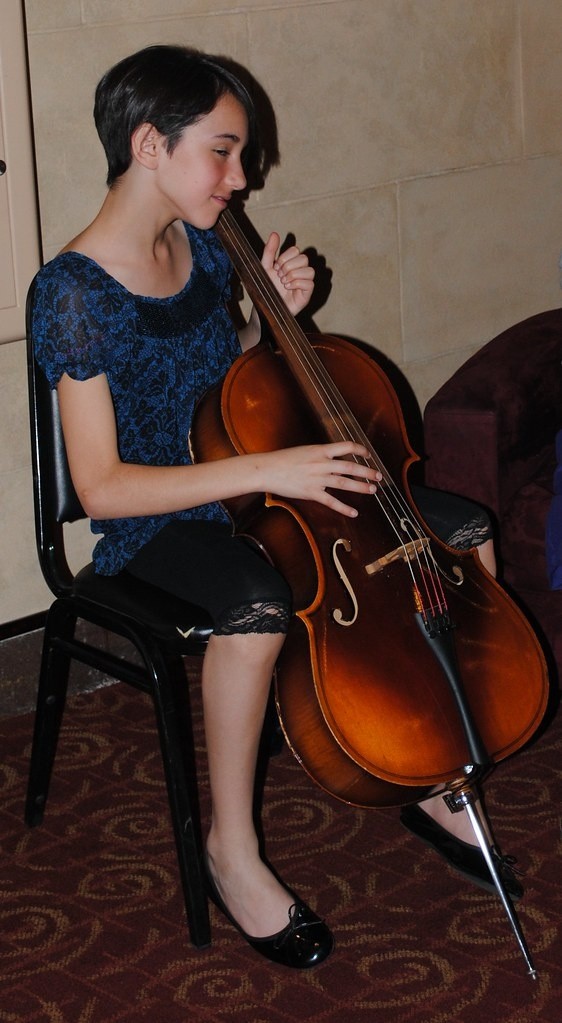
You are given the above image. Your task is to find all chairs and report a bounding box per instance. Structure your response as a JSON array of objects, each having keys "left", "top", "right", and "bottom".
[{"left": 20, "top": 340, "right": 218, "bottom": 954}]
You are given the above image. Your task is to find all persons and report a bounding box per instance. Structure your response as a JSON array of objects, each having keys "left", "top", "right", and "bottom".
[{"left": 26, "top": 44, "right": 523, "bottom": 968}]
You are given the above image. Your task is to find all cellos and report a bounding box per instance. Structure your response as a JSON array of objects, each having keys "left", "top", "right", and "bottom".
[{"left": 188, "top": 206, "right": 553, "bottom": 987}]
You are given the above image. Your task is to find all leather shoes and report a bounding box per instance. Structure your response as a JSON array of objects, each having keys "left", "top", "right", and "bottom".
[
  {"left": 202, "top": 848, "right": 336, "bottom": 970},
  {"left": 399, "top": 803, "right": 527, "bottom": 902}
]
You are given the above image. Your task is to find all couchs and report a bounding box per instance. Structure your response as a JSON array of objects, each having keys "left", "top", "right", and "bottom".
[{"left": 423, "top": 308, "right": 562, "bottom": 677}]
